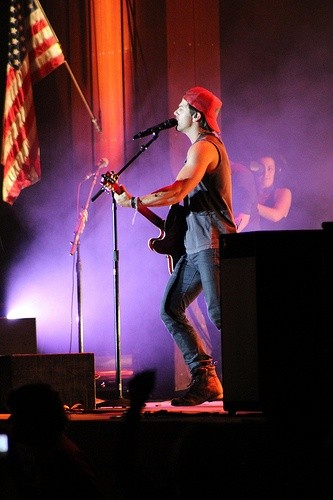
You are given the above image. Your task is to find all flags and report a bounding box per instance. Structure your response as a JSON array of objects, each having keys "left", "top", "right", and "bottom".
[{"left": 1, "top": 0, "right": 65, "bottom": 206}]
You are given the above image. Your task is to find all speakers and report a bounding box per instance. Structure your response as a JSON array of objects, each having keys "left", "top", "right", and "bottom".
[
  {"left": 218, "top": 228, "right": 333, "bottom": 415},
  {"left": 0, "top": 353, "right": 96, "bottom": 412}
]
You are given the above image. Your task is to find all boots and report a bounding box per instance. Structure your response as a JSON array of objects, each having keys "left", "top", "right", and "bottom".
[{"left": 171, "top": 364, "right": 223, "bottom": 406}]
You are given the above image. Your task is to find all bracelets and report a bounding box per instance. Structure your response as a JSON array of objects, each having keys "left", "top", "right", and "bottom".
[{"left": 130, "top": 197, "right": 136, "bottom": 208}]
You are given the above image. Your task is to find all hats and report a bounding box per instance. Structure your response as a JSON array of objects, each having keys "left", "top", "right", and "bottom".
[{"left": 183, "top": 87, "right": 223, "bottom": 135}]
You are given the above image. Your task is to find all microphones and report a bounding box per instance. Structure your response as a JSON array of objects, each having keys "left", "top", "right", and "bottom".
[
  {"left": 130, "top": 118, "right": 178, "bottom": 141},
  {"left": 86, "top": 157, "right": 109, "bottom": 180}
]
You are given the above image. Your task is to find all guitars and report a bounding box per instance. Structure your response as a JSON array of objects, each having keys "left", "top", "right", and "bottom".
[{"left": 100, "top": 169, "right": 185, "bottom": 275}]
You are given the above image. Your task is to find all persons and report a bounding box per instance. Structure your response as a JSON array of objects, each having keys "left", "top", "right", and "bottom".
[
  {"left": 114, "top": 88, "right": 238, "bottom": 407},
  {"left": 252, "top": 154, "right": 291, "bottom": 229},
  {"left": 197, "top": 161, "right": 260, "bottom": 387}
]
